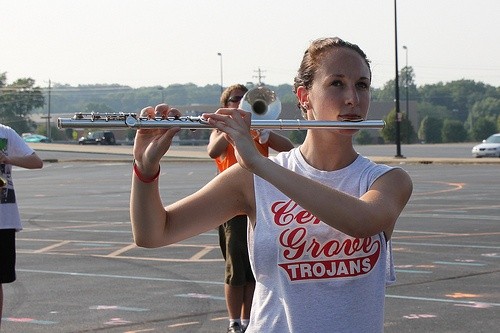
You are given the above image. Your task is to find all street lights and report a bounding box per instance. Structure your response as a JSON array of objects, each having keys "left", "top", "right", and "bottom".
[
  {"left": 217, "top": 52, "right": 223, "bottom": 93},
  {"left": 402, "top": 45, "right": 409, "bottom": 120}
]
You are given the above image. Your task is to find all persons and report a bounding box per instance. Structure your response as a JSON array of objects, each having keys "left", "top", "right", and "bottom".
[
  {"left": 206, "top": 84, "right": 295, "bottom": 333},
  {"left": 129, "top": 38, "right": 414, "bottom": 333},
  {"left": 0, "top": 124, "right": 44, "bottom": 326}
]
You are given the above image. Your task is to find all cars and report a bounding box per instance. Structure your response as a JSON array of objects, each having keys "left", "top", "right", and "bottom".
[
  {"left": 471, "top": 133, "right": 500, "bottom": 158},
  {"left": 78, "top": 131, "right": 117, "bottom": 145},
  {"left": 22, "top": 135, "right": 49, "bottom": 143}
]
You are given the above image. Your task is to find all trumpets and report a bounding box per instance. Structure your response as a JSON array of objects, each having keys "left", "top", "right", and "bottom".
[{"left": 237, "top": 85, "right": 281, "bottom": 131}]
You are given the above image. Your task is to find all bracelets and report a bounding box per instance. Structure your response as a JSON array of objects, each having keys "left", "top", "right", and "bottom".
[{"left": 133, "top": 159, "right": 160, "bottom": 182}]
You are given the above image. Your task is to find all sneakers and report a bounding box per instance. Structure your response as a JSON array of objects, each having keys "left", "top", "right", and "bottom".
[{"left": 227, "top": 322, "right": 247, "bottom": 333}]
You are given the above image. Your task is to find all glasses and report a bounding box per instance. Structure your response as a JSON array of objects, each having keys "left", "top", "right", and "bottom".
[{"left": 228, "top": 95, "right": 243, "bottom": 102}]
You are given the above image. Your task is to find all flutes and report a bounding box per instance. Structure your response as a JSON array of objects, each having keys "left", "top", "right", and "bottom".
[{"left": 57, "top": 111, "right": 387, "bottom": 131}]
